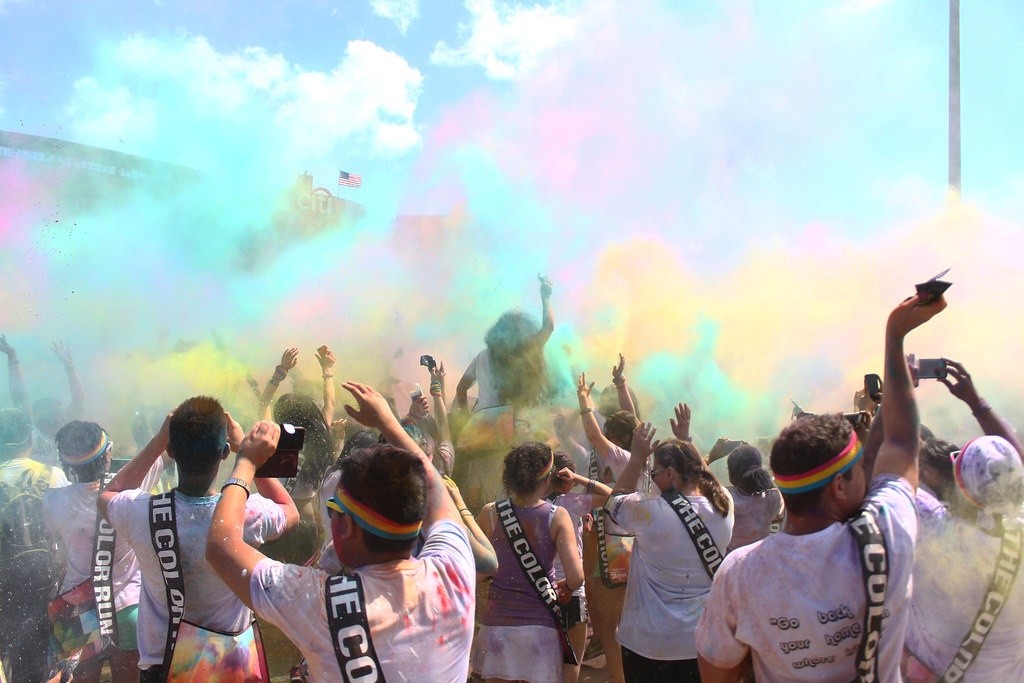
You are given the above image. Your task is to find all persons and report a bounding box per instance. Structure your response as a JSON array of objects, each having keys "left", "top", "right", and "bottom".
[
  {"left": 401, "top": 355, "right": 455, "bottom": 479},
  {"left": 455, "top": 273, "right": 651, "bottom": 683},
  {"left": 319, "top": 383, "right": 429, "bottom": 554},
  {"left": 696, "top": 292, "right": 1024, "bottom": 683},
  {"left": 0, "top": 334, "right": 175, "bottom": 683},
  {"left": 377, "top": 417, "right": 498, "bottom": 576},
  {"left": 603, "top": 402, "right": 735, "bottom": 683},
  {"left": 702, "top": 436, "right": 785, "bottom": 557},
  {"left": 97, "top": 396, "right": 298, "bottom": 683},
  {"left": 257, "top": 344, "right": 337, "bottom": 683},
  {"left": 206, "top": 381, "right": 476, "bottom": 683}
]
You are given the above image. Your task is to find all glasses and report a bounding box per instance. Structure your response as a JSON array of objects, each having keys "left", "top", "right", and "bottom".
[
  {"left": 105, "top": 441, "right": 115, "bottom": 458},
  {"left": 650, "top": 467, "right": 668, "bottom": 482},
  {"left": 950, "top": 451, "right": 960, "bottom": 462},
  {"left": 326, "top": 496, "right": 347, "bottom": 520}
]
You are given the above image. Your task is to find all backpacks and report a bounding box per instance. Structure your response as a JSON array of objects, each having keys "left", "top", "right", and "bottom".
[{"left": 4, "top": 470, "right": 61, "bottom": 605}]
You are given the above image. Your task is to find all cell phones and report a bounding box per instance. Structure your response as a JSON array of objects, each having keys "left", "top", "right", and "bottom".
[
  {"left": 276, "top": 427, "right": 306, "bottom": 451},
  {"left": 865, "top": 374, "right": 879, "bottom": 400},
  {"left": 726, "top": 441, "right": 744, "bottom": 450},
  {"left": 110, "top": 459, "right": 129, "bottom": 473},
  {"left": 914, "top": 358, "right": 947, "bottom": 378},
  {"left": 410, "top": 391, "right": 428, "bottom": 418},
  {"left": 842, "top": 414, "right": 863, "bottom": 429}
]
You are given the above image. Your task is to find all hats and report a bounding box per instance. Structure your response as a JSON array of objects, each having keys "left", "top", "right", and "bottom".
[
  {"left": 1, "top": 409, "right": 34, "bottom": 446},
  {"left": 953, "top": 435, "right": 1024, "bottom": 512}
]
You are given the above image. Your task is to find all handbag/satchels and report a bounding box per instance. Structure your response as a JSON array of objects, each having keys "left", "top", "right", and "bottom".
[
  {"left": 162, "top": 620, "right": 272, "bottom": 683},
  {"left": 599, "top": 534, "right": 636, "bottom": 589},
  {"left": 454, "top": 405, "right": 516, "bottom": 456},
  {"left": 47, "top": 578, "right": 119, "bottom": 669}
]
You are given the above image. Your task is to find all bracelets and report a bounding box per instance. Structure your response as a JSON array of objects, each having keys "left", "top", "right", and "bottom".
[
  {"left": 971, "top": 397, "right": 991, "bottom": 417},
  {"left": 580, "top": 408, "right": 592, "bottom": 414},
  {"left": 611, "top": 374, "right": 627, "bottom": 390},
  {"left": 322, "top": 373, "right": 333, "bottom": 378},
  {"left": 7, "top": 360, "right": 20, "bottom": 366},
  {"left": 565, "top": 580, "right": 583, "bottom": 592},
  {"left": 333, "top": 447, "right": 343, "bottom": 453},
  {"left": 269, "top": 365, "right": 288, "bottom": 387},
  {"left": 588, "top": 479, "right": 595, "bottom": 489},
  {"left": 459, "top": 509, "right": 474, "bottom": 517},
  {"left": 429, "top": 381, "right": 442, "bottom": 396},
  {"left": 221, "top": 477, "right": 250, "bottom": 501},
  {"left": 686, "top": 436, "right": 694, "bottom": 443}
]
